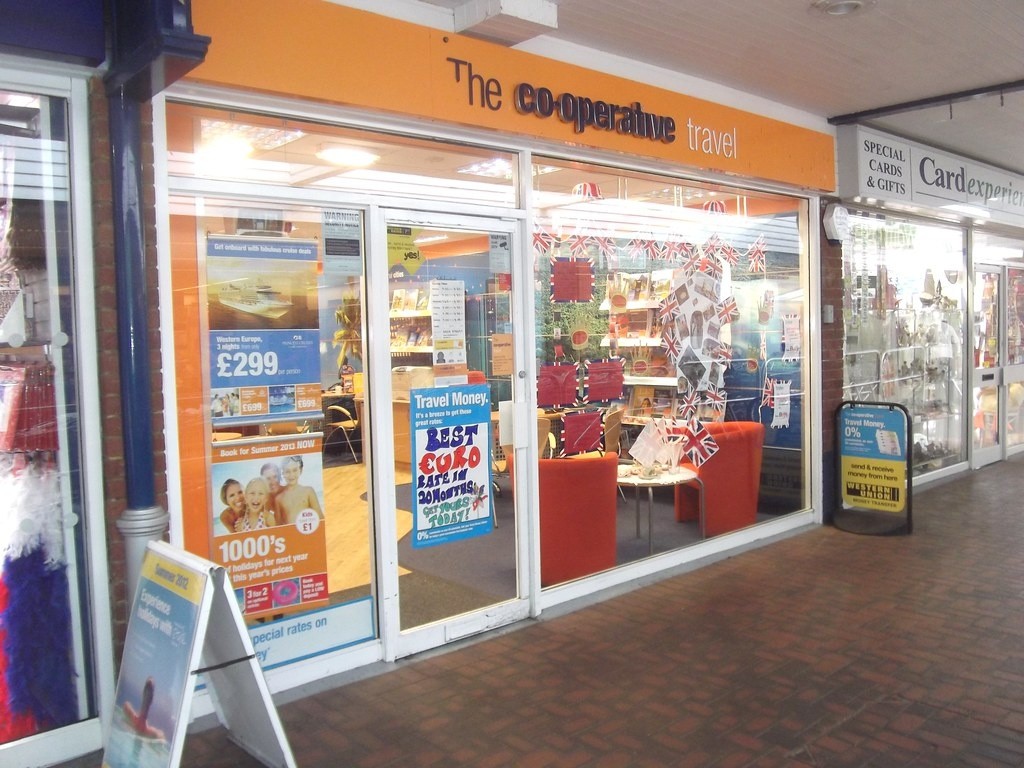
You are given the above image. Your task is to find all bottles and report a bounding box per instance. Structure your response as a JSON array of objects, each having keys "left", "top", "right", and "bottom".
[{"left": 390, "top": 316, "right": 430, "bottom": 339}]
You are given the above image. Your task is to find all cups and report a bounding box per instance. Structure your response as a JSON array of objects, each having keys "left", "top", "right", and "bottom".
[{"left": 335, "top": 386, "right": 342, "bottom": 394}]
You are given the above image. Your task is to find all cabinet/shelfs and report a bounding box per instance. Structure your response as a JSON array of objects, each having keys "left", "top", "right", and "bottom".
[
  {"left": 390, "top": 309, "right": 432, "bottom": 353},
  {"left": 600, "top": 269, "right": 680, "bottom": 458}
]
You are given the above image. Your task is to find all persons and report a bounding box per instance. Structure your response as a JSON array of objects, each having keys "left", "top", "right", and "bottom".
[
  {"left": 221, "top": 478, "right": 249, "bottom": 533},
  {"left": 260, "top": 463, "right": 286, "bottom": 525},
  {"left": 276, "top": 456, "right": 323, "bottom": 526},
  {"left": 236, "top": 478, "right": 276, "bottom": 532},
  {"left": 140, "top": 678, "right": 154, "bottom": 725},
  {"left": 923, "top": 313, "right": 960, "bottom": 414},
  {"left": 211, "top": 392, "right": 239, "bottom": 417},
  {"left": 637, "top": 398, "right": 651, "bottom": 417},
  {"left": 437, "top": 352, "right": 445, "bottom": 363}
]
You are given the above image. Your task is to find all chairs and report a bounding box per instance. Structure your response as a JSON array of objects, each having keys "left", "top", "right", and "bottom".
[
  {"left": 496, "top": 419, "right": 556, "bottom": 484},
  {"left": 595, "top": 407, "right": 627, "bottom": 456},
  {"left": 674, "top": 421, "right": 767, "bottom": 539},
  {"left": 507, "top": 452, "right": 617, "bottom": 590},
  {"left": 323, "top": 398, "right": 366, "bottom": 463}
]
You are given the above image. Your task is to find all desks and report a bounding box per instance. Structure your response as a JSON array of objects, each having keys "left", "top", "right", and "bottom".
[
  {"left": 321, "top": 392, "right": 355, "bottom": 441},
  {"left": 617, "top": 464, "right": 706, "bottom": 553}
]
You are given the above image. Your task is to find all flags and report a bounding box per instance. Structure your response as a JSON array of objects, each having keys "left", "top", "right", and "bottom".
[
  {"left": 704, "top": 383, "right": 726, "bottom": 411},
  {"left": 749, "top": 233, "right": 769, "bottom": 272},
  {"left": 702, "top": 253, "right": 723, "bottom": 281},
  {"left": 533, "top": 233, "right": 695, "bottom": 262},
  {"left": 718, "top": 341, "right": 733, "bottom": 370},
  {"left": 715, "top": 296, "right": 739, "bottom": 326},
  {"left": 659, "top": 328, "right": 683, "bottom": 362},
  {"left": 682, "top": 245, "right": 701, "bottom": 279},
  {"left": 628, "top": 412, "right": 719, "bottom": 469},
  {"left": 658, "top": 292, "right": 681, "bottom": 326},
  {"left": 761, "top": 377, "right": 787, "bottom": 408},
  {"left": 701, "top": 232, "right": 722, "bottom": 259},
  {"left": 678, "top": 386, "right": 703, "bottom": 419},
  {"left": 720, "top": 243, "right": 742, "bottom": 267}
]
